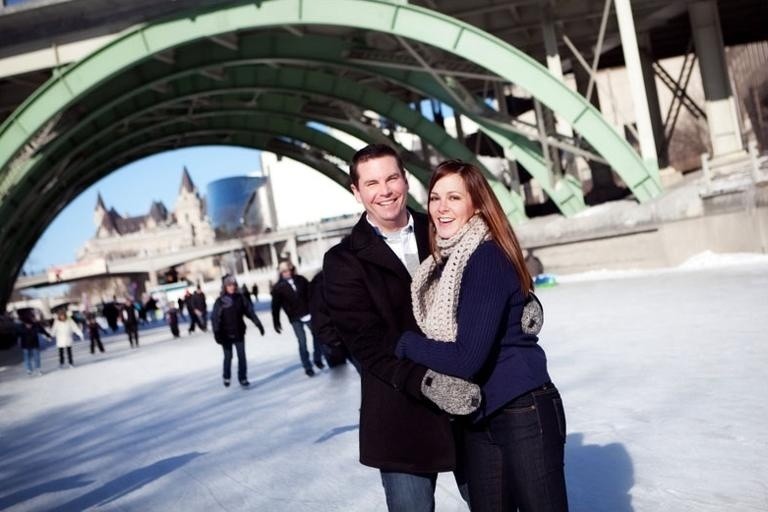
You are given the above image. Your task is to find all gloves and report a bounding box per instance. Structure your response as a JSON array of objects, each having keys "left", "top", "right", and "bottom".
[
  {"left": 521, "top": 294, "right": 543, "bottom": 335},
  {"left": 422, "top": 368, "right": 482, "bottom": 416}
]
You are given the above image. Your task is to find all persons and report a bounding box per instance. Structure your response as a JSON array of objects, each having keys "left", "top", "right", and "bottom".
[
  {"left": 321, "top": 144, "right": 544, "bottom": 511},
  {"left": 273, "top": 261, "right": 324, "bottom": 375},
  {"left": 18, "top": 274, "right": 264, "bottom": 387},
  {"left": 362, "top": 159, "right": 569, "bottom": 510}
]
